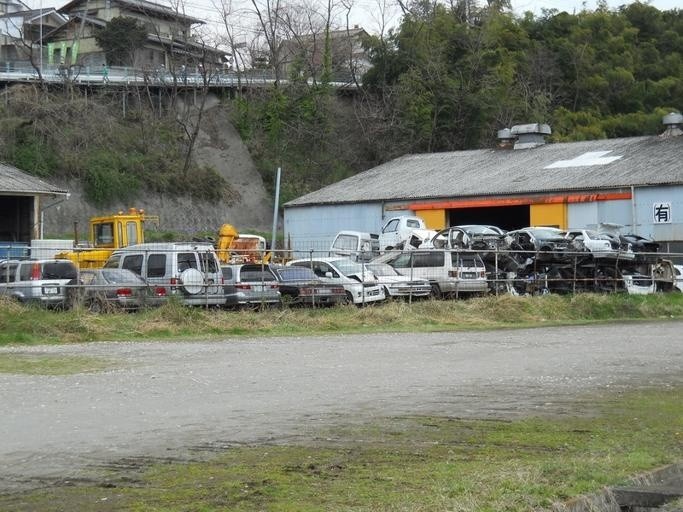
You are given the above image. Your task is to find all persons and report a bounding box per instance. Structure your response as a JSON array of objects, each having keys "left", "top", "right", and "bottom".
[{"left": 102, "top": 63, "right": 110, "bottom": 83}]
